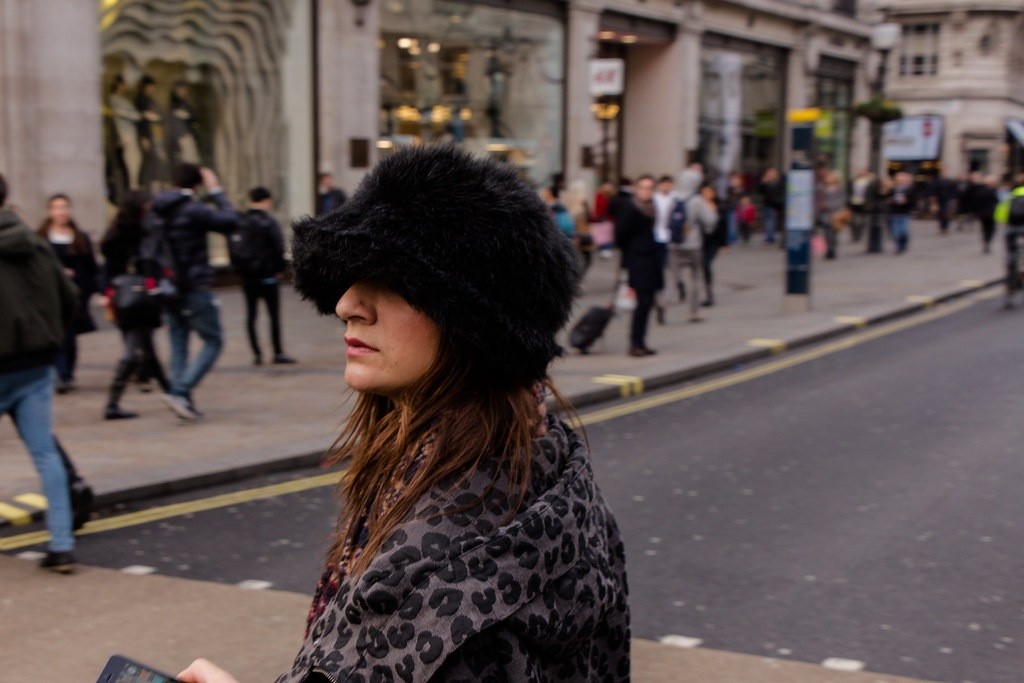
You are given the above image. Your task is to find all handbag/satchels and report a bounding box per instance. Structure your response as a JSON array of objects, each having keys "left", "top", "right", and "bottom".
[{"left": 112, "top": 275, "right": 163, "bottom": 330}]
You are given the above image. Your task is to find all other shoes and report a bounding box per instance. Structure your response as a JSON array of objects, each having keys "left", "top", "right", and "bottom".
[
  {"left": 629, "top": 346, "right": 656, "bottom": 357},
  {"left": 39, "top": 550, "right": 75, "bottom": 573},
  {"left": 272, "top": 353, "right": 296, "bottom": 364},
  {"left": 164, "top": 394, "right": 198, "bottom": 421},
  {"left": 68, "top": 482, "right": 92, "bottom": 532}
]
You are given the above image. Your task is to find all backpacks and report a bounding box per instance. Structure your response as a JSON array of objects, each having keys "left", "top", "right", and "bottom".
[{"left": 665, "top": 194, "right": 696, "bottom": 243}]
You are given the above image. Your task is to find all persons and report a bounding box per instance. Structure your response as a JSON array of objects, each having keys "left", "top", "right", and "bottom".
[
  {"left": 173, "top": 141, "right": 633, "bottom": 683},
  {"left": 995, "top": 173, "right": 1024, "bottom": 310},
  {"left": 99, "top": 160, "right": 349, "bottom": 423},
  {"left": 545, "top": 163, "right": 754, "bottom": 358},
  {"left": 759, "top": 151, "right": 997, "bottom": 259},
  {"left": 0, "top": 173, "right": 98, "bottom": 571}
]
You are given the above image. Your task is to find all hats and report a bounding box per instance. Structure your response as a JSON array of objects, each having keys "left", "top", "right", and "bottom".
[{"left": 292, "top": 147, "right": 579, "bottom": 386}]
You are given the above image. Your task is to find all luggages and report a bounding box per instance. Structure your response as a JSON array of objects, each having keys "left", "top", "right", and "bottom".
[{"left": 568, "top": 283, "right": 623, "bottom": 348}]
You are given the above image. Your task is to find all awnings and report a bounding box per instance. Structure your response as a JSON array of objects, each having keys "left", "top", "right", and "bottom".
[{"left": 1007, "top": 120, "right": 1024, "bottom": 146}]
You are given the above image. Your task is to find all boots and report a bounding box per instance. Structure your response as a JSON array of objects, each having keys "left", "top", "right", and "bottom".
[{"left": 104, "top": 384, "right": 138, "bottom": 418}]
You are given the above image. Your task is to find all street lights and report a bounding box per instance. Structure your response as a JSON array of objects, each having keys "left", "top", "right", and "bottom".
[{"left": 867, "top": 24, "right": 903, "bottom": 251}]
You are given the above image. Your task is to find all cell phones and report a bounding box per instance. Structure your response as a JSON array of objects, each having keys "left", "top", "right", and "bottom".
[{"left": 96, "top": 655, "right": 185, "bottom": 683}]
